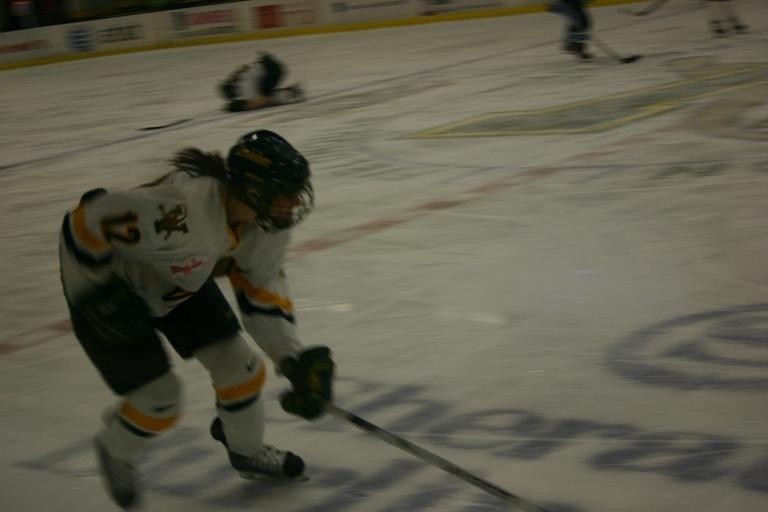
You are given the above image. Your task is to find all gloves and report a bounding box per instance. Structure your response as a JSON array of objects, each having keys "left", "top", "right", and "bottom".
[{"left": 279, "top": 344, "right": 335, "bottom": 421}]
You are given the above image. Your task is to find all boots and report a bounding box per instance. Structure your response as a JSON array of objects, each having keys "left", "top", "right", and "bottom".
[
  {"left": 209, "top": 417, "right": 308, "bottom": 479},
  {"left": 94, "top": 434, "right": 137, "bottom": 507}
]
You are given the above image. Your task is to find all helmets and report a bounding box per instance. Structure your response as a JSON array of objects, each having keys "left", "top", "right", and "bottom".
[{"left": 232, "top": 129, "right": 310, "bottom": 219}]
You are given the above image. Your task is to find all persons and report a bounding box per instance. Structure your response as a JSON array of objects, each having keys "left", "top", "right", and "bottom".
[
  {"left": 214, "top": 50, "right": 303, "bottom": 116},
  {"left": 698, "top": 1, "right": 755, "bottom": 38},
  {"left": 545, "top": 0, "right": 601, "bottom": 62},
  {"left": 57, "top": 126, "right": 336, "bottom": 512}
]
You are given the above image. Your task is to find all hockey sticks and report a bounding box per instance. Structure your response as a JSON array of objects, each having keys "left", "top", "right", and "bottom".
[
  {"left": 135, "top": 106, "right": 227, "bottom": 131},
  {"left": 587, "top": 35, "right": 643, "bottom": 64}
]
